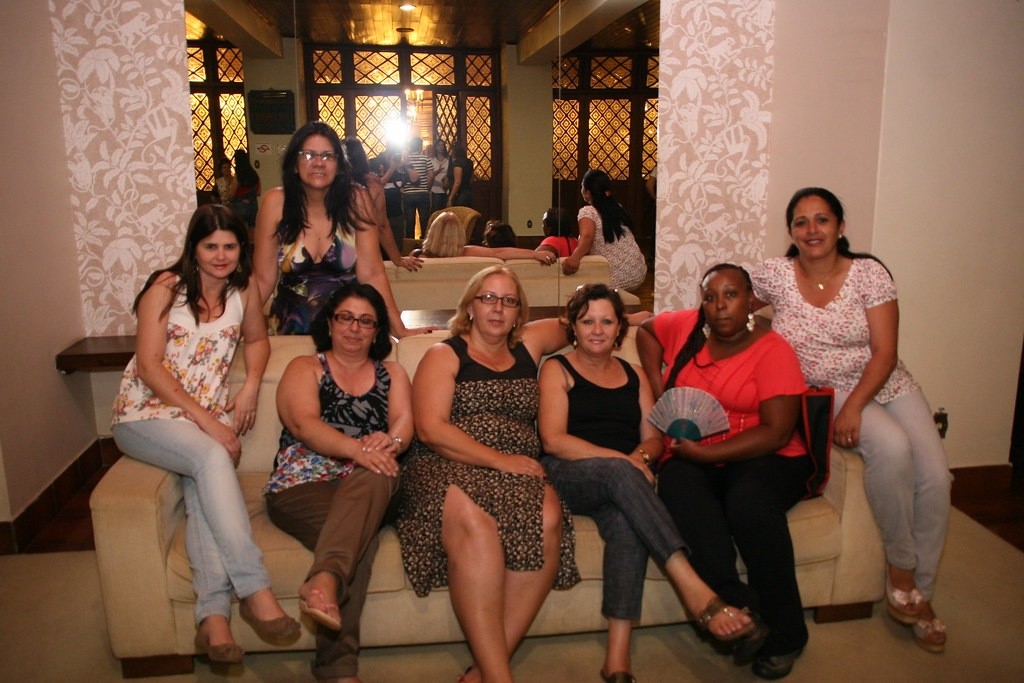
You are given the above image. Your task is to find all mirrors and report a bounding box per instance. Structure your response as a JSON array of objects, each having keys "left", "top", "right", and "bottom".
[{"left": 181, "top": 0, "right": 658, "bottom": 305}]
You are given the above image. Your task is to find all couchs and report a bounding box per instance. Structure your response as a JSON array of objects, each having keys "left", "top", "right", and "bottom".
[
  {"left": 89, "top": 327, "right": 892, "bottom": 679},
  {"left": 383, "top": 256, "right": 640, "bottom": 310}
]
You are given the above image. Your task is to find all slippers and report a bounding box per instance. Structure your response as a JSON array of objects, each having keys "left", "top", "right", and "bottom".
[
  {"left": 687, "top": 596, "right": 756, "bottom": 642},
  {"left": 296, "top": 582, "right": 341, "bottom": 630}
]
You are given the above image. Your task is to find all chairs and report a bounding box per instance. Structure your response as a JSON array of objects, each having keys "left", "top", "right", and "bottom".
[{"left": 402, "top": 206, "right": 482, "bottom": 256}]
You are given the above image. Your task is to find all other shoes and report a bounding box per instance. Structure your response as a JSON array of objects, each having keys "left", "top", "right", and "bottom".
[
  {"left": 753, "top": 642, "right": 802, "bottom": 678},
  {"left": 239, "top": 597, "right": 301, "bottom": 647},
  {"left": 193, "top": 629, "right": 245, "bottom": 664},
  {"left": 600, "top": 666, "right": 637, "bottom": 683}
]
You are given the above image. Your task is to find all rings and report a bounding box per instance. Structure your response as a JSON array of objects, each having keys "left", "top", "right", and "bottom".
[
  {"left": 847, "top": 438, "right": 852, "bottom": 441},
  {"left": 245, "top": 416, "right": 251, "bottom": 419},
  {"left": 546, "top": 256, "right": 550, "bottom": 260}
]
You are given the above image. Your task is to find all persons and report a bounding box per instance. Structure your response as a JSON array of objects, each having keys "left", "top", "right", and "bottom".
[
  {"left": 211, "top": 150, "right": 262, "bottom": 228},
  {"left": 750, "top": 187, "right": 954, "bottom": 653},
  {"left": 110, "top": 204, "right": 301, "bottom": 663},
  {"left": 252, "top": 121, "right": 407, "bottom": 341},
  {"left": 396, "top": 265, "right": 655, "bottom": 683},
  {"left": 340, "top": 135, "right": 424, "bottom": 272},
  {"left": 367, "top": 136, "right": 473, "bottom": 261},
  {"left": 536, "top": 283, "right": 756, "bottom": 683},
  {"left": 409, "top": 212, "right": 558, "bottom": 267},
  {"left": 483, "top": 220, "right": 517, "bottom": 248},
  {"left": 635, "top": 263, "right": 810, "bottom": 680},
  {"left": 562, "top": 169, "right": 648, "bottom": 293},
  {"left": 535, "top": 207, "right": 579, "bottom": 258},
  {"left": 261, "top": 281, "right": 415, "bottom": 683}
]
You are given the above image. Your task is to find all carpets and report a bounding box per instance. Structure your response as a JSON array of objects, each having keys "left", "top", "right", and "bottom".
[{"left": 0, "top": 505, "right": 1024, "bottom": 683}]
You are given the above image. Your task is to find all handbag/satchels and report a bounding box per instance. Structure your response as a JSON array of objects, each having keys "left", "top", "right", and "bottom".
[{"left": 801, "top": 385, "right": 834, "bottom": 498}]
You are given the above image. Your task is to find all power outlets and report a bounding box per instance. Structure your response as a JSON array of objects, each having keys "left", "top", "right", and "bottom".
[{"left": 933, "top": 407, "right": 948, "bottom": 439}]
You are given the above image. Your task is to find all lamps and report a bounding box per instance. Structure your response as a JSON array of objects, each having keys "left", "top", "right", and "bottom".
[{"left": 406, "top": 89, "right": 424, "bottom": 123}]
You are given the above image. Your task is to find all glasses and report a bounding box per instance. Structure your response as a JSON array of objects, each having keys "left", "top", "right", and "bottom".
[
  {"left": 475, "top": 293, "right": 520, "bottom": 308},
  {"left": 330, "top": 313, "right": 378, "bottom": 329},
  {"left": 298, "top": 150, "right": 339, "bottom": 161}
]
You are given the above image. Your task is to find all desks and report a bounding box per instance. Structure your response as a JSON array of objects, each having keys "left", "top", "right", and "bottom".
[{"left": 55, "top": 334, "right": 136, "bottom": 375}]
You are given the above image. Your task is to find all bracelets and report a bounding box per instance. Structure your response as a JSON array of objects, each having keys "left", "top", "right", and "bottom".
[
  {"left": 639, "top": 447, "right": 649, "bottom": 466},
  {"left": 393, "top": 437, "right": 402, "bottom": 448}
]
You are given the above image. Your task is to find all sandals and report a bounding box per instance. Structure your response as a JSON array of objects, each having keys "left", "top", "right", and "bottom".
[
  {"left": 886, "top": 560, "right": 922, "bottom": 623},
  {"left": 912, "top": 602, "right": 947, "bottom": 653}
]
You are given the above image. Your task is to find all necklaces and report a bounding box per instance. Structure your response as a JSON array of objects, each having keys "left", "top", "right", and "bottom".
[{"left": 818, "top": 283, "right": 824, "bottom": 290}]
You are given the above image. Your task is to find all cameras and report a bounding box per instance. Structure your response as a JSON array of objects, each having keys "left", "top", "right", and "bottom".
[{"left": 393, "top": 153, "right": 402, "bottom": 163}]
